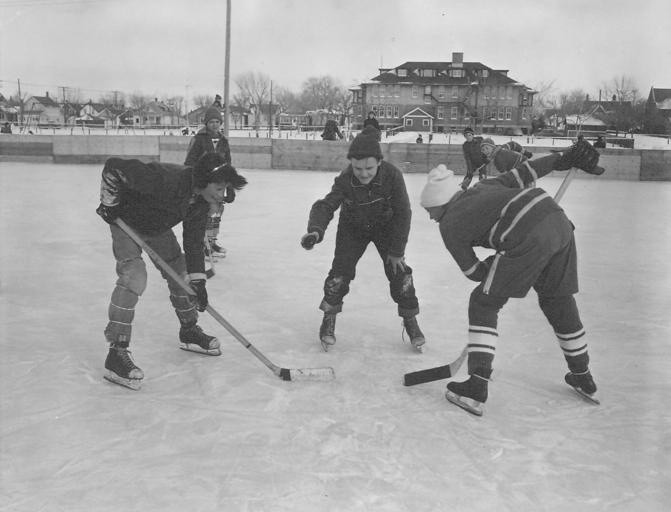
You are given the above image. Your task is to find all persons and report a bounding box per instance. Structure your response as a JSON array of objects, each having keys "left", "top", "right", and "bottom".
[
  {"left": 592, "top": 136, "right": 606, "bottom": 148},
  {"left": 318, "top": 116, "right": 343, "bottom": 142},
  {"left": 461, "top": 127, "right": 488, "bottom": 189},
  {"left": 477, "top": 136, "right": 532, "bottom": 174},
  {"left": 0, "top": 122, "right": 13, "bottom": 134},
  {"left": 300, "top": 129, "right": 427, "bottom": 347},
  {"left": 418, "top": 139, "right": 597, "bottom": 404},
  {"left": 95, "top": 151, "right": 249, "bottom": 381},
  {"left": 182, "top": 105, "right": 232, "bottom": 255},
  {"left": 212, "top": 94, "right": 228, "bottom": 114},
  {"left": 362, "top": 110, "right": 379, "bottom": 132}
]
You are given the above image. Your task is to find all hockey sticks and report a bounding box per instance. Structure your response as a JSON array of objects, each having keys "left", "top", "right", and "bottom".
[
  {"left": 405, "top": 167, "right": 578, "bottom": 385},
  {"left": 113, "top": 217, "right": 334, "bottom": 382}
]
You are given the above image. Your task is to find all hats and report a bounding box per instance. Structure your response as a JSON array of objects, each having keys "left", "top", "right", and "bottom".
[
  {"left": 347, "top": 132, "right": 384, "bottom": 159},
  {"left": 205, "top": 107, "right": 223, "bottom": 125},
  {"left": 462, "top": 125, "right": 474, "bottom": 134},
  {"left": 480, "top": 138, "right": 496, "bottom": 148},
  {"left": 421, "top": 165, "right": 462, "bottom": 209}
]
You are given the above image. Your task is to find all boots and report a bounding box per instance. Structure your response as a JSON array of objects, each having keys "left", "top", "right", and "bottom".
[
  {"left": 320, "top": 312, "right": 336, "bottom": 344},
  {"left": 404, "top": 316, "right": 426, "bottom": 346}
]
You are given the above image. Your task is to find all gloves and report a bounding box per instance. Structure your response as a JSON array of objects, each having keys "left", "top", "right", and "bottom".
[
  {"left": 97, "top": 203, "right": 119, "bottom": 224},
  {"left": 188, "top": 280, "right": 208, "bottom": 313},
  {"left": 566, "top": 139, "right": 599, "bottom": 170}
]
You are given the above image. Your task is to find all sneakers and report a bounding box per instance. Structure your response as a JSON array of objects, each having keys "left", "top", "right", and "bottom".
[
  {"left": 179, "top": 323, "right": 218, "bottom": 349},
  {"left": 106, "top": 342, "right": 144, "bottom": 380},
  {"left": 447, "top": 374, "right": 489, "bottom": 402},
  {"left": 566, "top": 371, "right": 595, "bottom": 393}
]
